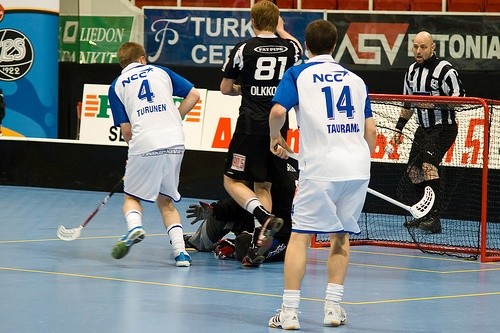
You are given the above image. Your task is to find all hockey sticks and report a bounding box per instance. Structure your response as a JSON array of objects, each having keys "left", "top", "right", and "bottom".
[
  {"left": 56, "top": 174, "right": 125, "bottom": 241},
  {"left": 274, "top": 143, "right": 435, "bottom": 219}
]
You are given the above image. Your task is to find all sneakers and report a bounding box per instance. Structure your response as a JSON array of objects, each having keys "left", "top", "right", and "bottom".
[
  {"left": 267, "top": 239, "right": 286, "bottom": 259},
  {"left": 404, "top": 217, "right": 428, "bottom": 229},
  {"left": 419, "top": 217, "right": 443, "bottom": 234},
  {"left": 268, "top": 309, "right": 300, "bottom": 330},
  {"left": 242, "top": 245, "right": 265, "bottom": 268},
  {"left": 250, "top": 214, "right": 283, "bottom": 255},
  {"left": 173, "top": 250, "right": 192, "bottom": 267},
  {"left": 323, "top": 305, "right": 347, "bottom": 326},
  {"left": 170, "top": 235, "right": 193, "bottom": 248},
  {"left": 111, "top": 226, "right": 146, "bottom": 259}
]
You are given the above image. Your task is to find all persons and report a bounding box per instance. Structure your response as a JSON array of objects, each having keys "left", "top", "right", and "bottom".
[
  {"left": 220, "top": 0, "right": 303, "bottom": 269},
  {"left": 108, "top": 42, "right": 200, "bottom": 267},
  {"left": 170, "top": 172, "right": 297, "bottom": 263},
  {"left": 268, "top": 19, "right": 376, "bottom": 329},
  {"left": 392, "top": 31, "right": 466, "bottom": 234}
]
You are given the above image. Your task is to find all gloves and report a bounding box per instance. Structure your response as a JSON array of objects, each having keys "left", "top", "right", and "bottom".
[
  {"left": 215, "top": 238, "right": 235, "bottom": 259},
  {"left": 186, "top": 202, "right": 214, "bottom": 225}
]
